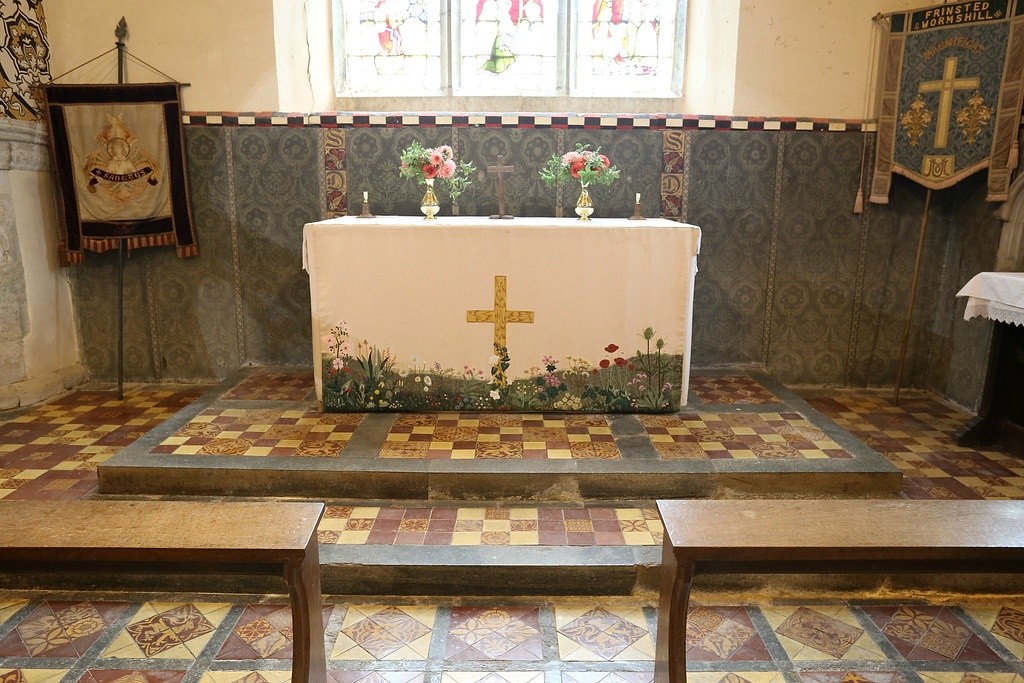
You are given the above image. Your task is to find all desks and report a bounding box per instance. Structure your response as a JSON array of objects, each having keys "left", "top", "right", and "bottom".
[
  {"left": 950, "top": 268, "right": 1024, "bottom": 462},
  {"left": 654, "top": 490, "right": 1024, "bottom": 683},
  {"left": 300, "top": 214, "right": 703, "bottom": 416},
  {"left": 0, "top": 498, "right": 332, "bottom": 683}
]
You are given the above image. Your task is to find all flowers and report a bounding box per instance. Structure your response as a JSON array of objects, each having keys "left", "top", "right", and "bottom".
[
  {"left": 538, "top": 141, "right": 622, "bottom": 188},
  {"left": 397, "top": 138, "right": 477, "bottom": 204}
]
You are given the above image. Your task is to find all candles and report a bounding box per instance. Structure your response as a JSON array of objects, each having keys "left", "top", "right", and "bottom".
[
  {"left": 362, "top": 190, "right": 368, "bottom": 203},
  {"left": 635, "top": 192, "right": 642, "bottom": 204}
]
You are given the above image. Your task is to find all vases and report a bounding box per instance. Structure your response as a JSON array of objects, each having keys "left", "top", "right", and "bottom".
[
  {"left": 418, "top": 178, "right": 440, "bottom": 220},
  {"left": 573, "top": 180, "right": 595, "bottom": 221}
]
